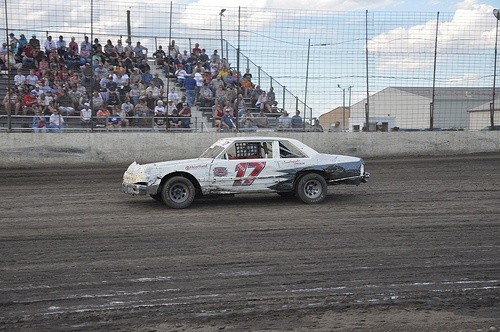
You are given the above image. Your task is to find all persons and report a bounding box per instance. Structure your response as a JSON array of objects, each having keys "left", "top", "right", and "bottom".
[
  {"left": 183, "top": 72, "right": 196, "bottom": 106},
  {"left": 130, "top": 68, "right": 142, "bottom": 89},
  {"left": 242, "top": 77, "right": 252, "bottom": 98},
  {"left": 80, "top": 102, "right": 96, "bottom": 128},
  {"left": 32, "top": 109, "right": 48, "bottom": 133},
  {"left": 230, "top": 143, "right": 264, "bottom": 160},
  {"left": 0, "top": 32, "right": 303, "bottom": 132},
  {"left": 105, "top": 76, "right": 120, "bottom": 102},
  {"left": 210, "top": 75, "right": 224, "bottom": 97},
  {"left": 49, "top": 109, "right": 65, "bottom": 133},
  {"left": 310, "top": 119, "right": 323, "bottom": 132},
  {"left": 134, "top": 41, "right": 147, "bottom": 60},
  {"left": 327, "top": 121, "right": 342, "bottom": 132},
  {"left": 255, "top": 91, "right": 272, "bottom": 113},
  {"left": 374, "top": 122, "right": 384, "bottom": 132}
]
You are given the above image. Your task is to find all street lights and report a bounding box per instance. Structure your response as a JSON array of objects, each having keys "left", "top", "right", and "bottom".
[
  {"left": 219, "top": 8, "right": 227, "bottom": 60},
  {"left": 337, "top": 84, "right": 345, "bottom": 131}
]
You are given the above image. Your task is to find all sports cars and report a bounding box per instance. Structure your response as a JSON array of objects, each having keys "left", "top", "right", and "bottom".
[{"left": 121, "top": 136, "right": 371, "bottom": 210}]
